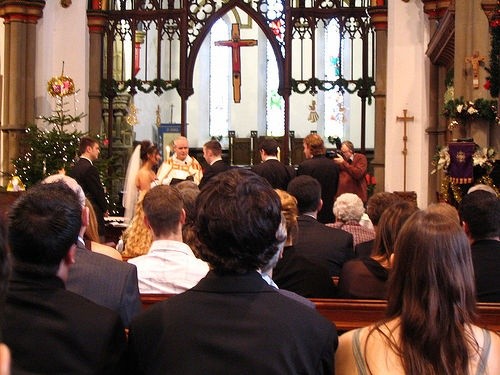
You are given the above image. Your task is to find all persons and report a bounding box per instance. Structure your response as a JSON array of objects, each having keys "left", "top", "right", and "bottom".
[
  {"left": 334, "top": 208, "right": 500, "bottom": 375},
  {"left": 125, "top": 168, "right": 338, "bottom": 375},
  {"left": 0, "top": 136, "right": 500, "bottom": 375}
]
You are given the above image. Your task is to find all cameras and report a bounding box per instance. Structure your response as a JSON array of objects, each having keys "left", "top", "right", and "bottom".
[{"left": 325, "top": 150, "right": 344, "bottom": 159}]
